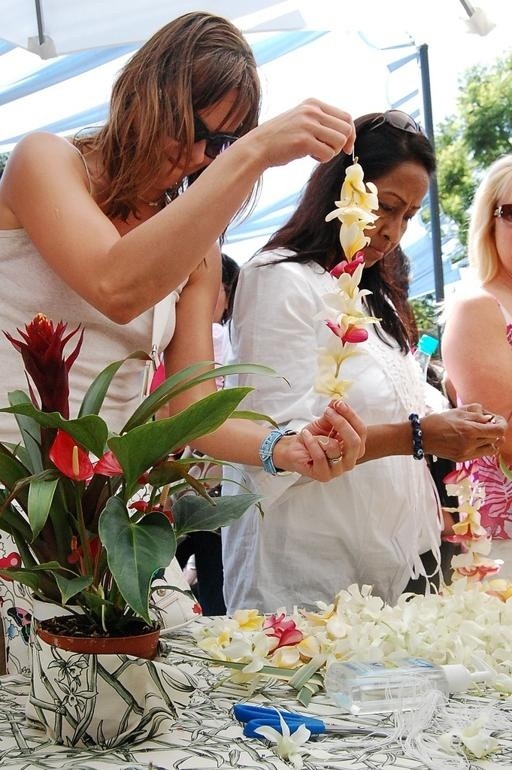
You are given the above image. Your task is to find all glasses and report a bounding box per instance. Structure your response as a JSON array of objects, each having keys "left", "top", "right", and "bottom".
[
  {"left": 495, "top": 204, "right": 512, "bottom": 221},
  {"left": 169, "top": 109, "right": 240, "bottom": 159},
  {"left": 366, "top": 109, "right": 423, "bottom": 136}
]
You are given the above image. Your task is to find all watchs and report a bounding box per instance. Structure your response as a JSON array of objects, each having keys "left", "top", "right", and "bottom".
[{"left": 259, "top": 427, "right": 299, "bottom": 478}]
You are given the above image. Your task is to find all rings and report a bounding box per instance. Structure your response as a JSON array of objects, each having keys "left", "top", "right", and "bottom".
[{"left": 329, "top": 457, "right": 343, "bottom": 464}]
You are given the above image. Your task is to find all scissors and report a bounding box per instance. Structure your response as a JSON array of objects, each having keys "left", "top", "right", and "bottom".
[{"left": 233, "top": 704, "right": 388, "bottom": 738}]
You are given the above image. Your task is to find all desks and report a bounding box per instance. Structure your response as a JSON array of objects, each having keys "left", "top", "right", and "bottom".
[{"left": 2, "top": 588, "right": 512, "bottom": 770}]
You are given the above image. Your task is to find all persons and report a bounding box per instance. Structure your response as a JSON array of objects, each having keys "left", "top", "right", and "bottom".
[
  {"left": 0, "top": 10, "right": 368, "bottom": 560},
  {"left": 160, "top": 252, "right": 241, "bottom": 616},
  {"left": 219, "top": 109, "right": 507, "bottom": 621},
  {"left": 441, "top": 152, "right": 512, "bottom": 583},
  {"left": 376, "top": 244, "right": 459, "bottom": 538}
]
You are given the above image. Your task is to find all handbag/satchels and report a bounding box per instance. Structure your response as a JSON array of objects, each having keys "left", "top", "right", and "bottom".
[{"left": 149, "top": 556, "right": 203, "bottom": 636}]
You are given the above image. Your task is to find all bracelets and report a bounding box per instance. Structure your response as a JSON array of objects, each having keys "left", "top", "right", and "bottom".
[{"left": 408, "top": 413, "right": 425, "bottom": 460}]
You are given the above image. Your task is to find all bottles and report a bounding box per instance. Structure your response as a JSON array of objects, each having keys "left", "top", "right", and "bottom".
[{"left": 413, "top": 330, "right": 441, "bottom": 378}]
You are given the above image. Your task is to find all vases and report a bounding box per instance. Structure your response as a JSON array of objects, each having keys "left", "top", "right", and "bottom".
[{"left": 33, "top": 616, "right": 161, "bottom": 658}]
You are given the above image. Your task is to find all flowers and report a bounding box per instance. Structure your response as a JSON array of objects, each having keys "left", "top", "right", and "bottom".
[
  {"left": 321, "top": 139, "right": 380, "bottom": 399},
  {"left": 0, "top": 313, "right": 292, "bottom": 636}
]
